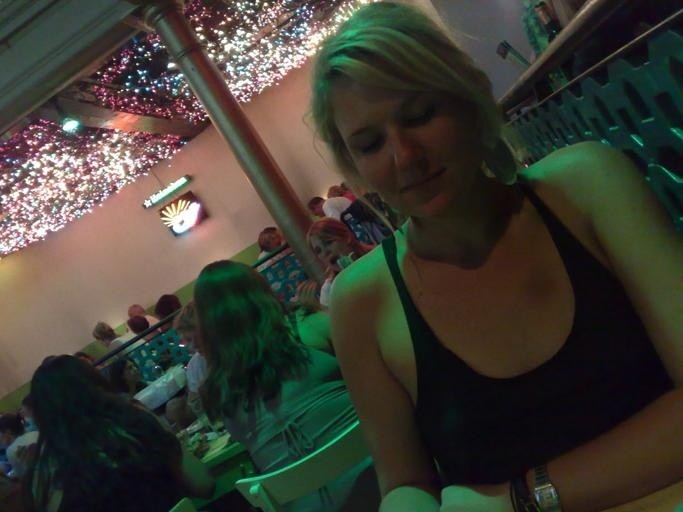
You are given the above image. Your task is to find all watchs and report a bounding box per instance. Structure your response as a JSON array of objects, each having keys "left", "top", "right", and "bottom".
[{"left": 532, "top": 459, "right": 563, "bottom": 512}]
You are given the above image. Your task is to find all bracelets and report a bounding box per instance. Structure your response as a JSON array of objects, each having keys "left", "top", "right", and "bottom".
[{"left": 509, "top": 469, "right": 539, "bottom": 512}]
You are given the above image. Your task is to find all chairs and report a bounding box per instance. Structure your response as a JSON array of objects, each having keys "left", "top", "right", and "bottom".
[{"left": 231, "top": 421, "right": 373, "bottom": 510}]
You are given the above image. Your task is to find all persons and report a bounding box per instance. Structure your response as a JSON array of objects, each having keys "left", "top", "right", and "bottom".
[
  {"left": 0, "top": 181, "right": 380, "bottom": 512},
  {"left": 302, "top": 2, "right": 683, "bottom": 512}
]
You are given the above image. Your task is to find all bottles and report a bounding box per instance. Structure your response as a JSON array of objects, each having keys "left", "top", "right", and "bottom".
[{"left": 531, "top": 8, "right": 563, "bottom": 44}]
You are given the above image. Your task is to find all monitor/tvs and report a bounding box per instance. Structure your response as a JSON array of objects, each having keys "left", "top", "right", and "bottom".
[{"left": 156, "top": 191, "right": 209, "bottom": 238}]
